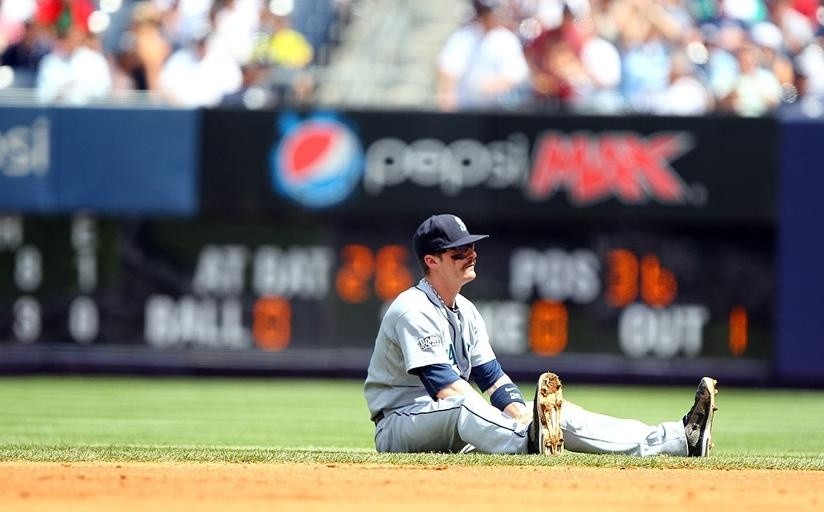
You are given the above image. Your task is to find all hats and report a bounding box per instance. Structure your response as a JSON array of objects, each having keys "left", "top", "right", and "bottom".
[{"left": 412, "top": 214, "right": 491, "bottom": 260}]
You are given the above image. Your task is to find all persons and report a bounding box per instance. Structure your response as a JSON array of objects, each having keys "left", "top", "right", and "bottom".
[{"left": 362, "top": 214, "right": 719, "bottom": 457}]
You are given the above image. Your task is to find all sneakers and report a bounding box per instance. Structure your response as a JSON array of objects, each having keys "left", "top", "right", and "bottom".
[
  {"left": 683, "top": 377, "right": 718, "bottom": 457},
  {"left": 528, "top": 371, "right": 564, "bottom": 454}
]
